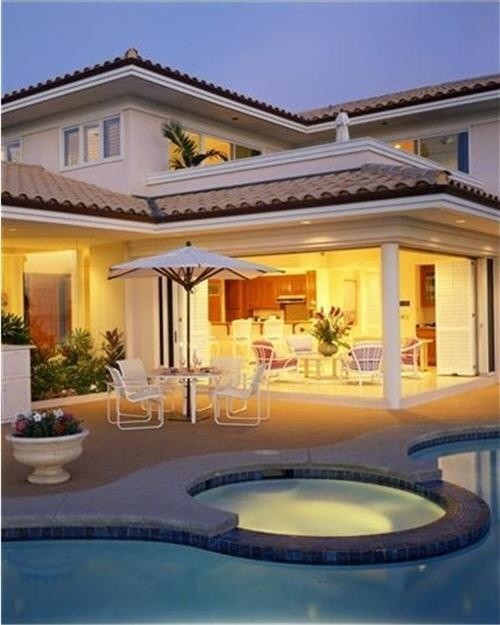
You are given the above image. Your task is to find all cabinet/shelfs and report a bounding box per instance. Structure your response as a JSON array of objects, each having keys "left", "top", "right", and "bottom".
[
  {"left": 419, "top": 266, "right": 435, "bottom": 307},
  {"left": 224, "top": 270, "right": 316, "bottom": 321}
]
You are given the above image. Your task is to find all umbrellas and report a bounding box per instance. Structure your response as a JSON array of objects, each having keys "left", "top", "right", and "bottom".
[{"left": 108, "top": 241, "right": 286, "bottom": 418}]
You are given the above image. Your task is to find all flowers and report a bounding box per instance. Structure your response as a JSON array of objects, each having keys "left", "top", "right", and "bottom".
[
  {"left": 308, "top": 305, "right": 354, "bottom": 349},
  {"left": 11, "top": 409, "right": 84, "bottom": 437}
]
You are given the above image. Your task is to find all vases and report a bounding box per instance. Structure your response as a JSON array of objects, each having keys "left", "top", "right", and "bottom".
[{"left": 5, "top": 428, "right": 90, "bottom": 485}]
